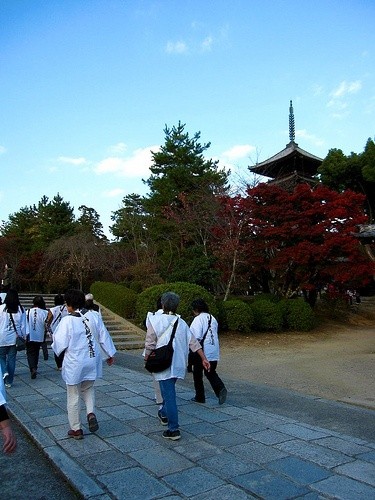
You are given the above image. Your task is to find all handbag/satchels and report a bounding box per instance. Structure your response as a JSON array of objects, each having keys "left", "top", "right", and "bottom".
[
  {"left": 98, "top": 343, "right": 110, "bottom": 362},
  {"left": 16, "top": 337, "right": 26, "bottom": 351},
  {"left": 145, "top": 345, "right": 174, "bottom": 373},
  {"left": 186, "top": 340, "right": 204, "bottom": 373}
]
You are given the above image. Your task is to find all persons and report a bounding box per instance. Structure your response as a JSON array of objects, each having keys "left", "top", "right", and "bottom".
[
  {"left": 0, "top": 363, "right": 17, "bottom": 454},
  {"left": 143, "top": 292, "right": 210, "bottom": 440},
  {"left": 0, "top": 289, "right": 102, "bottom": 387},
  {"left": 189, "top": 298, "right": 227, "bottom": 405},
  {"left": 51, "top": 289, "right": 116, "bottom": 439}
]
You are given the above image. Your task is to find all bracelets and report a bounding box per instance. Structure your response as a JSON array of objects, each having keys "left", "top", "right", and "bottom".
[{"left": 144, "top": 359, "right": 147, "bottom": 361}]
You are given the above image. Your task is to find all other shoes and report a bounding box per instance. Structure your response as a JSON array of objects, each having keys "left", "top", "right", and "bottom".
[
  {"left": 191, "top": 398, "right": 205, "bottom": 403},
  {"left": 218, "top": 388, "right": 227, "bottom": 404},
  {"left": 31, "top": 369, "right": 37, "bottom": 379},
  {"left": 2, "top": 373, "right": 9, "bottom": 380},
  {"left": 5, "top": 383, "right": 11, "bottom": 387}
]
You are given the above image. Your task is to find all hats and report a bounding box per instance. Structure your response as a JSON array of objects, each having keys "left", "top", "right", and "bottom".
[
  {"left": 161, "top": 291, "right": 179, "bottom": 308},
  {"left": 85, "top": 293, "right": 94, "bottom": 301}
]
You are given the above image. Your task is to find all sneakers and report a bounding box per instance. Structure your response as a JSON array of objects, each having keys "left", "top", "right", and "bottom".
[
  {"left": 157, "top": 409, "right": 168, "bottom": 425},
  {"left": 87, "top": 413, "right": 99, "bottom": 432},
  {"left": 162, "top": 429, "right": 181, "bottom": 440},
  {"left": 68, "top": 429, "right": 84, "bottom": 440}
]
